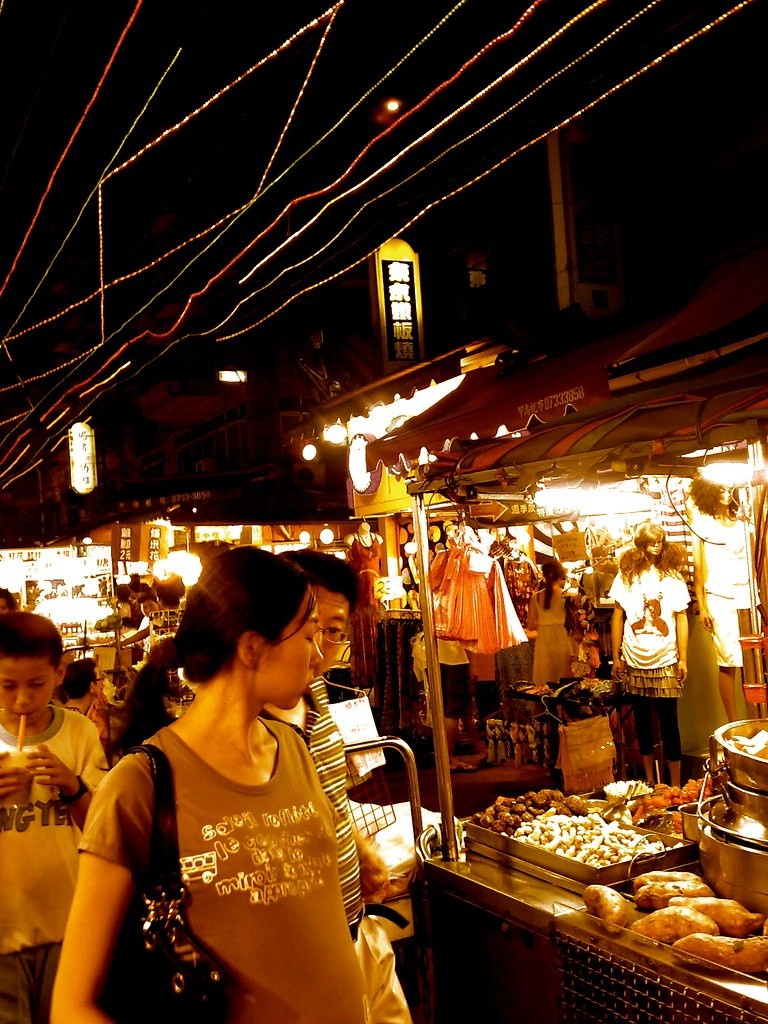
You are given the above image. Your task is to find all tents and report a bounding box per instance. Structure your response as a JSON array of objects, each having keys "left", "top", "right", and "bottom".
[{"left": 367, "top": 259, "right": 767, "bottom": 859}]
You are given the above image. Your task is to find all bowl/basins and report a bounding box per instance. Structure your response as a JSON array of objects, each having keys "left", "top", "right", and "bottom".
[
  {"left": 678, "top": 801, "right": 701, "bottom": 841},
  {"left": 603, "top": 780, "right": 654, "bottom": 804}
]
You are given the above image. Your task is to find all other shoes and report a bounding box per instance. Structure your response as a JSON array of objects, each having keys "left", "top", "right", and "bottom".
[{"left": 449, "top": 759, "right": 478, "bottom": 772}]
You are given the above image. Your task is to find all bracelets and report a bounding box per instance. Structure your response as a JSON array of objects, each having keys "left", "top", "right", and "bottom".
[{"left": 57, "top": 774, "right": 89, "bottom": 804}]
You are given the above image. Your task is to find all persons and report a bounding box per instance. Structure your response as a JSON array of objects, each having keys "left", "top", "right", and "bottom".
[
  {"left": 528, "top": 559, "right": 577, "bottom": 687},
  {"left": 0, "top": 611, "right": 108, "bottom": 1024},
  {"left": 51, "top": 547, "right": 367, "bottom": 1024},
  {"left": 256, "top": 548, "right": 417, "bottom": 1024},
  {"left": 611, "top": 525, "right": 690, "bottom": 789},
  {"left": 691, "top": 463, "right": 762, "bottom": 724},
  {"left": 1, "top": 568, "right": 186, "bottom": 769}
]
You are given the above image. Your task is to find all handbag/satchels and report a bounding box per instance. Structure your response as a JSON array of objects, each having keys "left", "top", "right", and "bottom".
[{"left": 94, "top": 744, "right": 233, "bottom": 1024}]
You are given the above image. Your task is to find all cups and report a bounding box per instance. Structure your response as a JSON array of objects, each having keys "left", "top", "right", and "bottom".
[
  {"left": 0, "top": 750, "right": 36, "bottom": 809},
  {"left": 667, "top": 805, "right": 682, "bottom": 834}
]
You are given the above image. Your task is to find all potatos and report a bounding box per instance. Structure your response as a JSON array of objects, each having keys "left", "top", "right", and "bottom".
[{"left": 582, "top": 870, "right": 768, "bottom": 973}]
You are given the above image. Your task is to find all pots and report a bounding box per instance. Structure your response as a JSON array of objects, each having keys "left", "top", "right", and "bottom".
[{"left": 697, "top": 718, "right": 768, "bottom": 913}]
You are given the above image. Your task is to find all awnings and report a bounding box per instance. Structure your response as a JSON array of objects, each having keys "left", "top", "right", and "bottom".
[{"left": 280, "top": 341, "right": 500, "bottom": 443}]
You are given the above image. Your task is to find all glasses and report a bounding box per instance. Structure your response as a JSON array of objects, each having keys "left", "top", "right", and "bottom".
[
  {"left": 314, "top": 623, "right": 348, "bottom": 644},
  {"left": 96, "top": 677, "right": 104, "bottom": 682}
]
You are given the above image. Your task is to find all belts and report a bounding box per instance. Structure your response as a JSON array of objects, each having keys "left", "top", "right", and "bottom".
[{"left": 349, "top": 903, "right": 409, "bottom": 943}]
